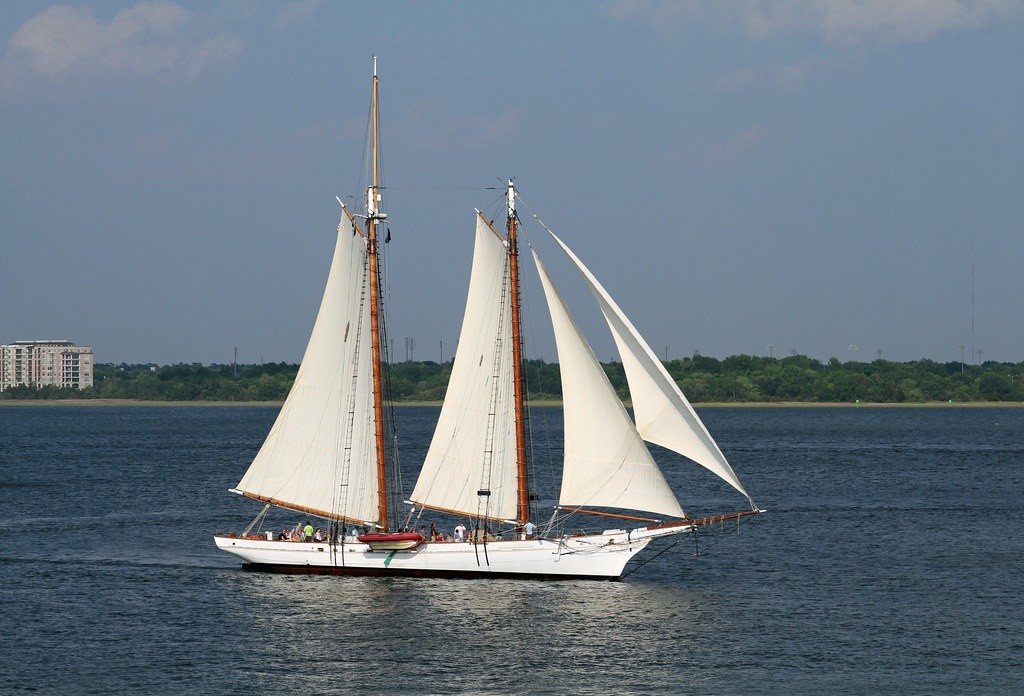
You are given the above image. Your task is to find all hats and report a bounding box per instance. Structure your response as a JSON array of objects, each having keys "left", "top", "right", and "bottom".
[{"left": 297, "top": 522, "right": 302, "bottom": 525}]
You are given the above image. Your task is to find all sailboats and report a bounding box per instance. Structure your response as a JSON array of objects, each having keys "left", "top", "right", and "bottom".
[{"left": 214, "top": 52, "right": 767, "bottom": 588}]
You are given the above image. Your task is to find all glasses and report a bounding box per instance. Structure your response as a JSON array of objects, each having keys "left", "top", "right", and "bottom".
[
  {"left": 324, "top": 530, "right": 326, "bottom": 531},
  {"left": 284, "top": 531, "right": 285, "bottom": 532}
]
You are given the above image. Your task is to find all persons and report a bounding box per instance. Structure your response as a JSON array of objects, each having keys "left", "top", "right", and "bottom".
[
  {"left": 512, "top": 519, "right": 537, "bottom": 541},
  {"left": 352, "top": 526, "right": 359, "bottom": 536},
  {"left": 454, "top": 522, "right": 466, "bottom": 543},
  {"left": 279, "top": 520, "right": 327, "bottom": 543},
  {"left": 420, "top": 520, "right": 444, "bottom": 543}
]
